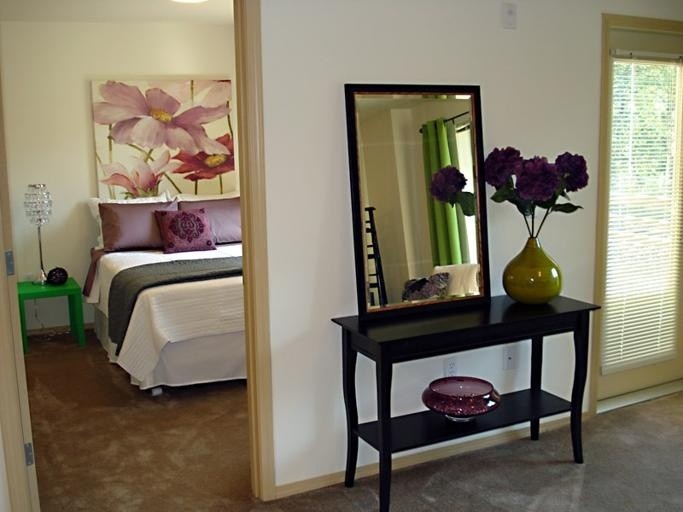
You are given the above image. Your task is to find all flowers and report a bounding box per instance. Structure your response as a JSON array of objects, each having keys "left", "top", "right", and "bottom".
[
  {"left": 482, "top": 141, "right": 590, "bottom": 237},
  {"left": 427, "top": 164, "right": 476, "bottom": 218}
]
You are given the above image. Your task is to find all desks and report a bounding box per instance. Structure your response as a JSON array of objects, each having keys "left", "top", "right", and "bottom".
[
  {"left": 329, "top": 291, "right": 602, "bottom": 511},
  {"left": 18, "top": 276, "right": 85, "bottom": 353}
]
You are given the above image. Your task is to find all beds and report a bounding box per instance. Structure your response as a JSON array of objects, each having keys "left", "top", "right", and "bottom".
[{"left": 88, "top": 191, "right": 247, "bottom": 397}]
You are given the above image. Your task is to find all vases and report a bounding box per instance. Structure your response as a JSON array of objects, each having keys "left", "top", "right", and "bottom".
[{"left": 502, "top": 236, "right": 562, "bottom": 305}]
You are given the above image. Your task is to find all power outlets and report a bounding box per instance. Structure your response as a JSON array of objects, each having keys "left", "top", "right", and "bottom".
[
  {"left": 441, "top": 357, "right": 457, "bottom": 380},
  {"left": 503, "top": 344, "right": 520, "bottom": 370}
]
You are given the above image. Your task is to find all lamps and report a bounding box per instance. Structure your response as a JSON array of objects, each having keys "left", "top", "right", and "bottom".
[{"left": 23, "top": 183, "right": 54, "bottom": 285}]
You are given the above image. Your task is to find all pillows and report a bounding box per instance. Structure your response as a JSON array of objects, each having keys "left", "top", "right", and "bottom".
[
  {"left": 87, "top": 193, "right": 167, "bottom": 250},
  {"left": 99, "top": 201, "right": 179, "bottom": 252},
  {"left": 166, "top": 190, "right": 241, "bottom": 201},
  {"left": 178, "top": 195, "right": 241, "bottom": 244},
  {"left": 154, "top": 205, "right": 216, "bottom": 254}
]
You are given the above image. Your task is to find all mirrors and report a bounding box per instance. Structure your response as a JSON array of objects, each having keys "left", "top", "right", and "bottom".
[{"left": 343, "top": 83, "right": 491, "bottom": 324}]
